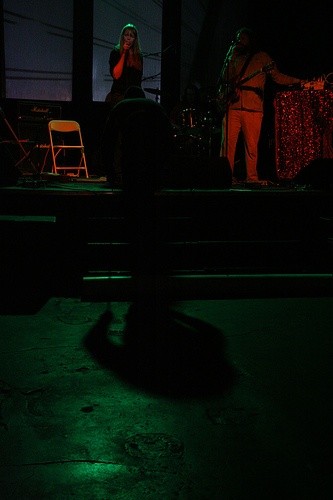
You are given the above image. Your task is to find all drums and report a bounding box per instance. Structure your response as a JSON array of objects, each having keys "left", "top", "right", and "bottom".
[
  {"left": 178, "top": 106, "right": 201, "bottom": 130},
  {"left": 178, "top": 133, "right": 208, "bottom": 150},
  {"left": 200, "top": 112, "right": 220, "bottom": 131}
]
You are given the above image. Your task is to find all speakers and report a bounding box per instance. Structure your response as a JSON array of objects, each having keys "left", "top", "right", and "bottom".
[
  {"left": 169, "top": 157, "right": 232, "bottom": 189},
  {"left": 11, "top": 121, "right": 66, "bottom": 177}
]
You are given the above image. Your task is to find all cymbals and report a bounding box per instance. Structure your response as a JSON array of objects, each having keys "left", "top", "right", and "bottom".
[{"left": 144, "top": 87, "right": 169, "bottom": 97}]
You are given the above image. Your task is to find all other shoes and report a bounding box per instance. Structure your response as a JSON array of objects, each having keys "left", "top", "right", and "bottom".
[
  {"left": 232, "top": 176, "right": 239, "bottom": 185},
  {"left": 247, "top": 177, "right": 259, "bottom": 183}
]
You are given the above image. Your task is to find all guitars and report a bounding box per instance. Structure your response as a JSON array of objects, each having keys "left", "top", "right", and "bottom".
[{"left": 218, "top": 60, "right": 276, "bottom": 111}]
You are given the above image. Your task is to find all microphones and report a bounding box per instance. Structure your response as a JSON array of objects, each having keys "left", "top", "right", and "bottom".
[{"left": 231, "top": 40, "right": 242, "bottom": 44}]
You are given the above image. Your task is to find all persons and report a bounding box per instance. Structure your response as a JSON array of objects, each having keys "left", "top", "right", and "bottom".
[
  {"left": 217, "top": 27, "right": 316, "bottom": 186},
  {"left": 106, "top": 23, "right": 144, "bottom": 179}
]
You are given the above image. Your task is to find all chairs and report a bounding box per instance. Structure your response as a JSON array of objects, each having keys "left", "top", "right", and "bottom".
[{"left": 46, "top": 118, "right": 89, "bottom": 180}]
